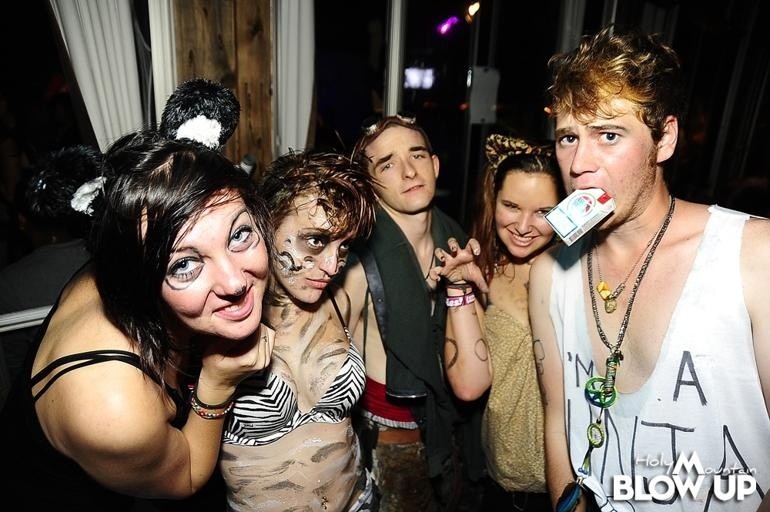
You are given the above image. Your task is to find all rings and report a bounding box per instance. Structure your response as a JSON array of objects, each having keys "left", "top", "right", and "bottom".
[{"left": 260, "top": 334, "right": 268, "bottom": 343}]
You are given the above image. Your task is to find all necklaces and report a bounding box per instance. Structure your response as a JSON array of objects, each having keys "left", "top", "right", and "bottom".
[
  {"left": 422, "top": 239, "right": 437, "bottom": 282},
  {"left": 555, "top": 191, "right": 674, "bottom": 512},
  {"left": 589, "top": 218, "right": 666, "bottom": 315}
]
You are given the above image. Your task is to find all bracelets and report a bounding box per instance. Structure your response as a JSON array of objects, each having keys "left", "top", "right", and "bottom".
[
  {"left": 186, "top": 382, "right": 235, "bottom": 420},
  {"left": 194, "top": 374, "right": 241, "bottom": 409},
  {"left": 445, "top": 283, "right": 472, "bottom": 297},
  {"left": 446, "top": 292, "right": 475, "bottom": 307}
]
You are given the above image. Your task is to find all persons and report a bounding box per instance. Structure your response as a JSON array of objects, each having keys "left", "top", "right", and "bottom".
[
  {"left": 350, "top": 108, "right": 473, "bottom": 512},
  {"left": 526, "top": 22, "right": 770, "bottom": 512},
  {"left": 214, "top": 146, "right": 383, "bottom": 511},
  {"left": 0, "top": 75, "right": 276, "bottom": 511},
  {"left": 429, "top": 133, "right": 566, "bottom": 511}
]
